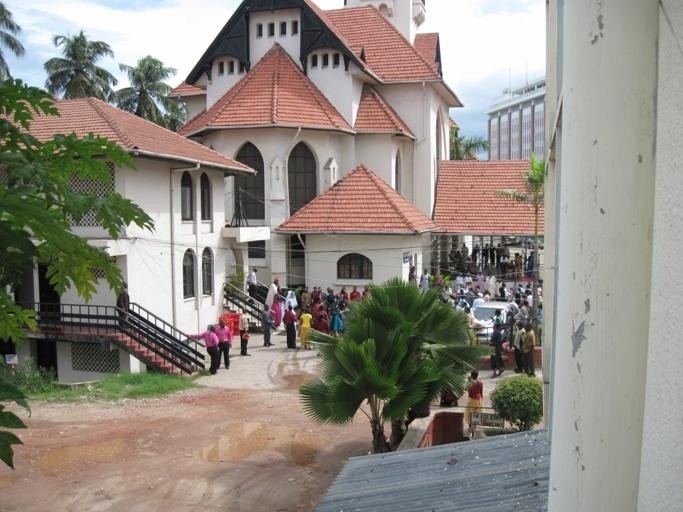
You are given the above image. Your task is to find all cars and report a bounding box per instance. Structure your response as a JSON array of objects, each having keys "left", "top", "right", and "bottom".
[
  {"left": 468, "top": 298, "right": 519, "bottom": 343},
  {"left": 504, "top": 235, "right": 544, "bottom": 249}
]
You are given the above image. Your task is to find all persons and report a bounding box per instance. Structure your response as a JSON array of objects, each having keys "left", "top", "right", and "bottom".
[
  {"left": 215, "top": 320, "right": 232, "bottom": 369},
  {"left": 117, "top": 281, "right": 129, "bottom": 332},
  {"left": 191, "top": 324, "right": 219, "bottom": 374},
  {"left": 405, "top": 242, "right": 543, "bottom": 433},
  {"left": 249, "top": 267, "right": 257, "bottom": 299},
  {"left": 238, "top": 309, "right": 251, "bottom": 356},
  {"left": 261, "top": 276, "right": 369, "bottom": 350}
]
